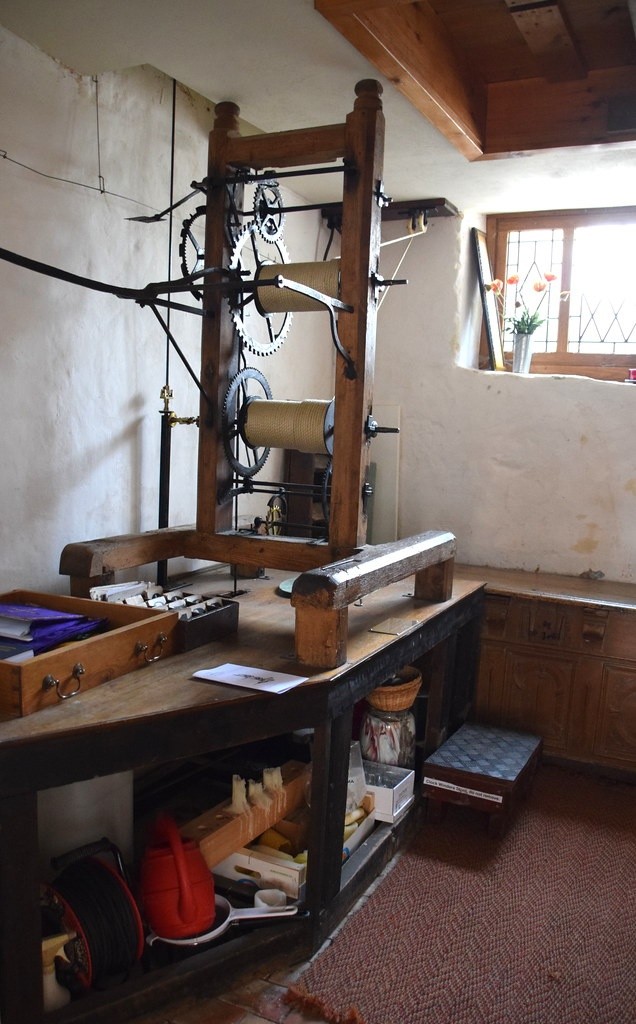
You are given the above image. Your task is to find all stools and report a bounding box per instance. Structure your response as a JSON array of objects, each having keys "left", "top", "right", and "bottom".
[{"left": 423, "top": 721, "right": 543, "bottom": 838}]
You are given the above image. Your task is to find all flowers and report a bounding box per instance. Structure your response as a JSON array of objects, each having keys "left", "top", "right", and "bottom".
[{"left": 489, "top": 271, "right": 557, "bottom": 335}]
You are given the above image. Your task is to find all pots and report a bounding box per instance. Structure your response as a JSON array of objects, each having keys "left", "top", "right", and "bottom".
[{"left": 147, "top": 892, "right": 299, "bottom": 948}]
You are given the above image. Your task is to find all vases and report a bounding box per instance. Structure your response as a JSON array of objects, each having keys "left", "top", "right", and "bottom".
[
  {"left": 360, "top": 706, "right": 416, "bottom": 769},
  {"left": 513, "top": 333, "right": 534, "bottom": 375}
]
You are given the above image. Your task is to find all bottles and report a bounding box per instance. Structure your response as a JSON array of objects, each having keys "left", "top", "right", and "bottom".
[{"left": 359, "top": 703, "right": 416, "bottom": 770}]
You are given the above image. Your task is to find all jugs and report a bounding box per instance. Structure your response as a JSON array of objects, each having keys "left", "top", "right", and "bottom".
[{"left": 139, "top": 814, "right": 217, "bottom": 937}]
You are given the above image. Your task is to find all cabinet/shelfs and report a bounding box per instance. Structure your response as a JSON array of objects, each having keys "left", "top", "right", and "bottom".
[{"left": 482, "top": 588, "right": 636, "bottom": 770}]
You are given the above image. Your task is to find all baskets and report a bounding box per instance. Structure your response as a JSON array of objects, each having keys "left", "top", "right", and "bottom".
[{"left": 366, "top": 666, "right": 422, "bottom": 712}]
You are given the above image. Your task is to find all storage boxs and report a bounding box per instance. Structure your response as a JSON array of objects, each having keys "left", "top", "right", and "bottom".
[
  {"left": 366, "top": 760, "right": 415, "bottom": 824},
  {"left": 209, "top": 807, "right": 374, "bottom": 900},
  {"left": 158, "top": 588, "right": 239, "bottom": 652},
  {"left": 0, "top": 589, "right": 178, "bottom": 716}
]
